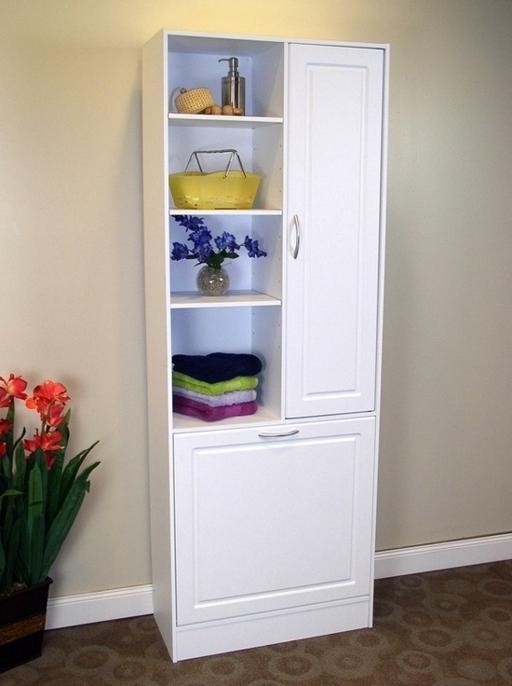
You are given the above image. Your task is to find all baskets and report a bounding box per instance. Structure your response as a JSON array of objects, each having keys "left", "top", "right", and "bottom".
[{"left": 169, "top": 150, "right": 261, "bottom": 209}]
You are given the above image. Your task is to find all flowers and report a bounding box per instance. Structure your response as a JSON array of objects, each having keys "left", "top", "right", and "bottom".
[
  {"left": 169, "top": 215, "right": 267, "bottom": 267},
  {"left": 0, "top": 372, "right": 101, "bottom": 592}
]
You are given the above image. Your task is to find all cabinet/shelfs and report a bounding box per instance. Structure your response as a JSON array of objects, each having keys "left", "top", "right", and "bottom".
[{"left": 138, "top": 27, "right": 392, "bottom": 665}]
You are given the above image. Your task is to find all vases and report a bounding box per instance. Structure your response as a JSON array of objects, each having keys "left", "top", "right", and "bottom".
[
  {"left": 196, "top": 264, "right": 231, "bottom": 297},
  {"left": 0, "top": 577, "right": 52, "bottom": 674}
]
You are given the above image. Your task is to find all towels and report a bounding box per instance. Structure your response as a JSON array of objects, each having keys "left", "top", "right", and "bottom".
[
  {"left": 172, "top": 352, "right": 262, "bottom": 383},
  {"left": 173, "top": 395, "right": 257, "bottom": 423},
  {"left": 172, "top": 386, "right": 257, "bottom": 407},
  {"left": 172, "top": 371, "right": 260, "bottom": 398}
]
